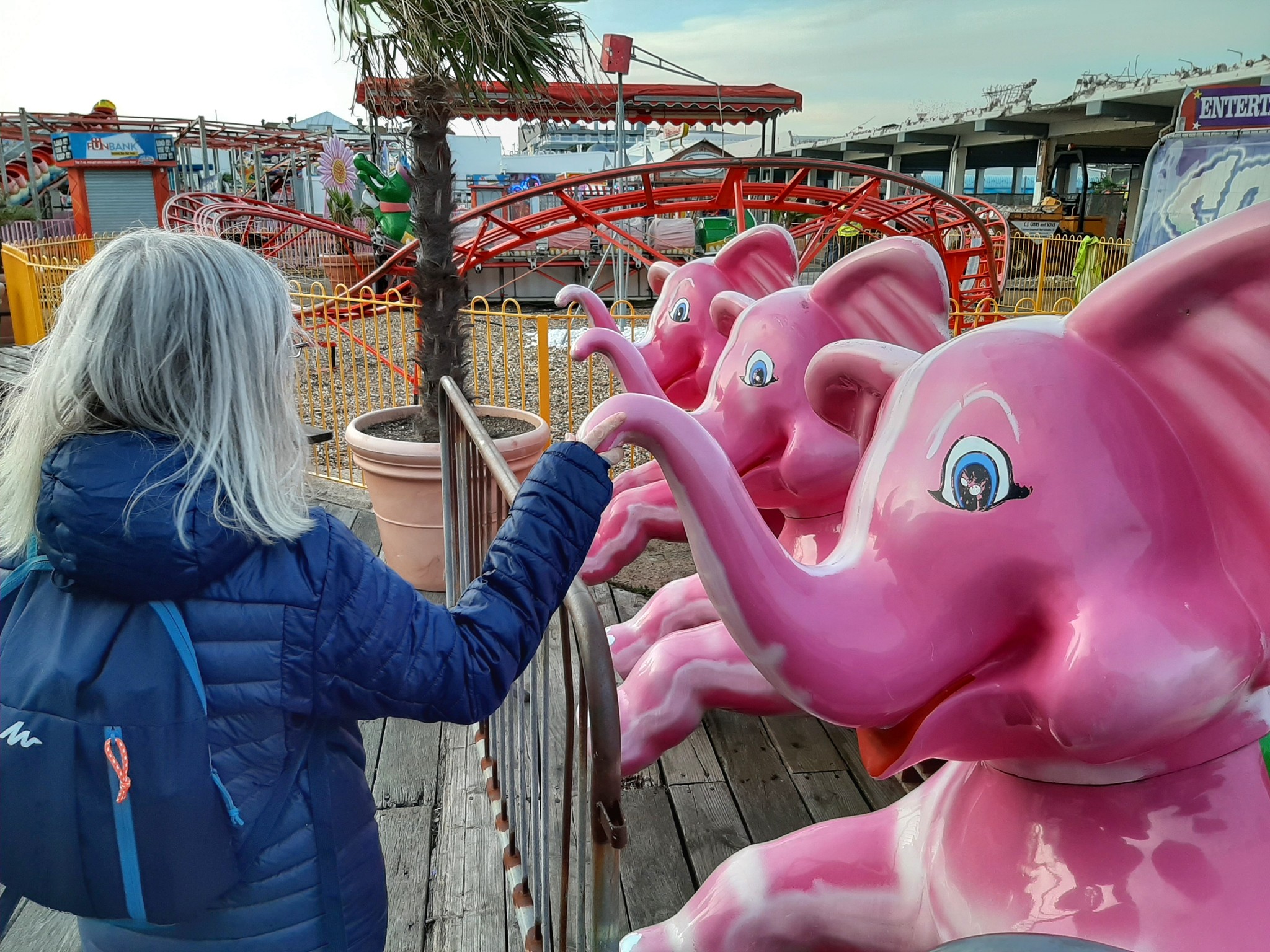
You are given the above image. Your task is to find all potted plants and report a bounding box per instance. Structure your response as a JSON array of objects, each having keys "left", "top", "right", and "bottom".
[
  {"left": 319, "top": 186, "right": 376, "bottom": 306},
  {"left": 327, "top": 0, "right": 592, "bottom": 592}
]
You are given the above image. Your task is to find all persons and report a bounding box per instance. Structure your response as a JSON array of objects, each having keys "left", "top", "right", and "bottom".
[
  {"left": 0, "top": 225, "right": 627, "bottom": 952},
  {"left": 822, "top": 203, "right": 864, "bottom": 269}
]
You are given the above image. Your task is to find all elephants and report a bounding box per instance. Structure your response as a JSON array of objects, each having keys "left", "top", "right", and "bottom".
[{"left": 553, "top": 222, "right": 1270, "bottom": 952}]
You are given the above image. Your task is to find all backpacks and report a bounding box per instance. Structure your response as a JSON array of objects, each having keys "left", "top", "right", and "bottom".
[{"left": 1, "top": 533, "right": 312, "bottom": 937}]
